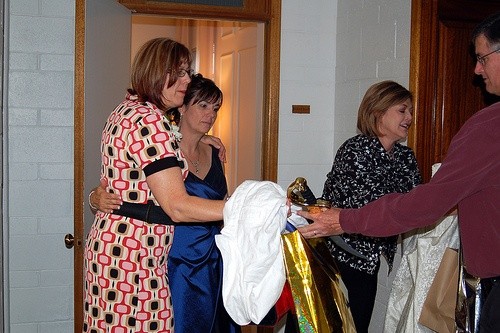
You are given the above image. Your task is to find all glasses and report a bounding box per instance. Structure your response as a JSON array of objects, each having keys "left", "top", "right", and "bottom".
[
  {"left": 477, "top": 49, "right": 500, "bottom": 65},
  {"left": 167, "top": 68, "right": 195, "bottom": 78}
]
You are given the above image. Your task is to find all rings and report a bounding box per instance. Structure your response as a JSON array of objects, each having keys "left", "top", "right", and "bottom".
[{"left": 314, "top": 231, "right": 316, "bottom": 236}]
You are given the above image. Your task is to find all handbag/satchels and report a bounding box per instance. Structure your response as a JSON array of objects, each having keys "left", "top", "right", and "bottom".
[
  {"left": 279, "top": 229, "right": 359, "bottom": 333},
  {"left": 417, "top": 223, "right": 464, "bottom": 333}
]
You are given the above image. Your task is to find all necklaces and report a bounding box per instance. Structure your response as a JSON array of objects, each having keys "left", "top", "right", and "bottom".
[{"left": 181, "top": 148, "right": 201, "bottom": 174}]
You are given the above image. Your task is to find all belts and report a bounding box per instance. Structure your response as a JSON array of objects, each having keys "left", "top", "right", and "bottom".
[{"left": 113, "top": 201, "right": 176, "bottom": 225}]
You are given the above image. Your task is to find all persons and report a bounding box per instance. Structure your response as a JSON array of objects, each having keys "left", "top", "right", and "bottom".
[
  {"left": 87, "top": 72, "right": 241, "bottom": 333},
  {"left": 293, "top": 20, "right": 499, "bottom": 333},
  {"left": 321, "top": 80, "right": 424, "bottom": 333},
  {"left": 82, "top": 37, "right": 292, "bottom": 333}
]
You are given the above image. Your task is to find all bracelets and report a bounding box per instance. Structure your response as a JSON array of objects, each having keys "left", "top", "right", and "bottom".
[{"left": 88, "top": 191, "right": 98, "bottom": 211}]
